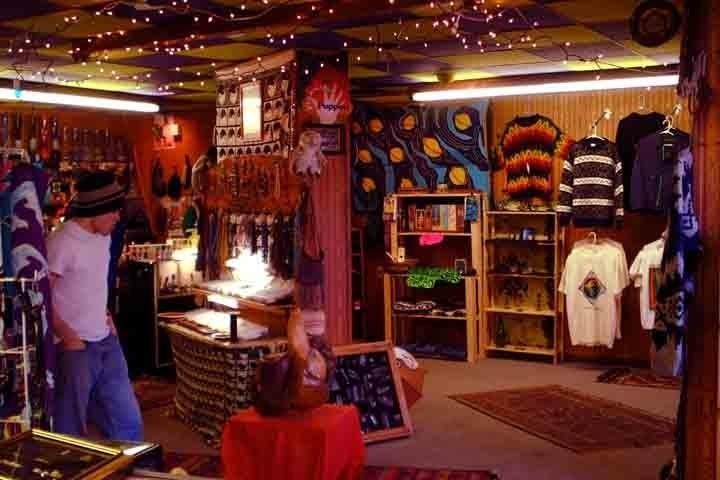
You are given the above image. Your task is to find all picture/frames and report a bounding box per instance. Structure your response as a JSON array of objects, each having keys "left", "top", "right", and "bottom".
[{"left": 331, "top": 339, "right": 414, "bottom": 443}]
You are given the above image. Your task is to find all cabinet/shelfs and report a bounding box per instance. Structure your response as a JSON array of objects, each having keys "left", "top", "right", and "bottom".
[{"left": 385, "top": 190, "right": 563, "bottom": 367}]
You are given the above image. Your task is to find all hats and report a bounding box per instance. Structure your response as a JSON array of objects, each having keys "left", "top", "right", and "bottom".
[{"left": 70, "top": 172, "right": 128, "bottom": 217}]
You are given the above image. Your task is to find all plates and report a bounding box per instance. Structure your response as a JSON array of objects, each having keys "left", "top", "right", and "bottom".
[
  {"left": 158, "top": 311, "right": 185, "bottom": 322},
  {"left": 212, "top": 332, "right": 230, "bottom": 339}
]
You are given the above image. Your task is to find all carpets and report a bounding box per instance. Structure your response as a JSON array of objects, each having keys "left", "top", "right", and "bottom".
[
  {"left": 444, "top": 363, "right": 685, "bottom": 456},
  {"left": 157, "top": 450, "right": 501, "bottom": 480}
]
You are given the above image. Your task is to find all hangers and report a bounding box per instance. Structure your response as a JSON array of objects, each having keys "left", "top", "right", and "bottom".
[
  {"left": 660, "top": 116, "right": 676, "bottom": 138},
  {"left": 634, "top": 90, "right": 655, "bottom": 114},
  {"left": 580, "top": 227, "right": 602, "bottom": 254},
  {"left": 586, "top": 123, "right": 605, "bottom": 143}
]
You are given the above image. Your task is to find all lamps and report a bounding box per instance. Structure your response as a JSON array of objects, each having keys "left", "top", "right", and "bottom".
[
  {"left": 0, "top": 87, "right": 160, "bottom": 113},
  {"left": 411, "top": 73, "right": 679, "bottom": 102}
]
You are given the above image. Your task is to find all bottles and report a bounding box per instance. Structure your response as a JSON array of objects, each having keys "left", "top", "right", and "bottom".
[{"left": 230, "top": 314, "right": 238, "bottom": 342}]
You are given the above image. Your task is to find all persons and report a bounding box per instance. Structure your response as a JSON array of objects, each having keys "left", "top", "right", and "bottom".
[{"left": 45, "top": 169, "right": 145, "bottom": 442}]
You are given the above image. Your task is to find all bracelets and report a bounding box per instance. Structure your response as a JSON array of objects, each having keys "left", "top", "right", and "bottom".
[{"left": 107, "top": 310, "right": 113, "bottom": 320}]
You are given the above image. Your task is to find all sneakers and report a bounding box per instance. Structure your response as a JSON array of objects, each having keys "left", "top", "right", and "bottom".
[{"left": 133, "top": 463, "right": 186, "bottom": 478}]
